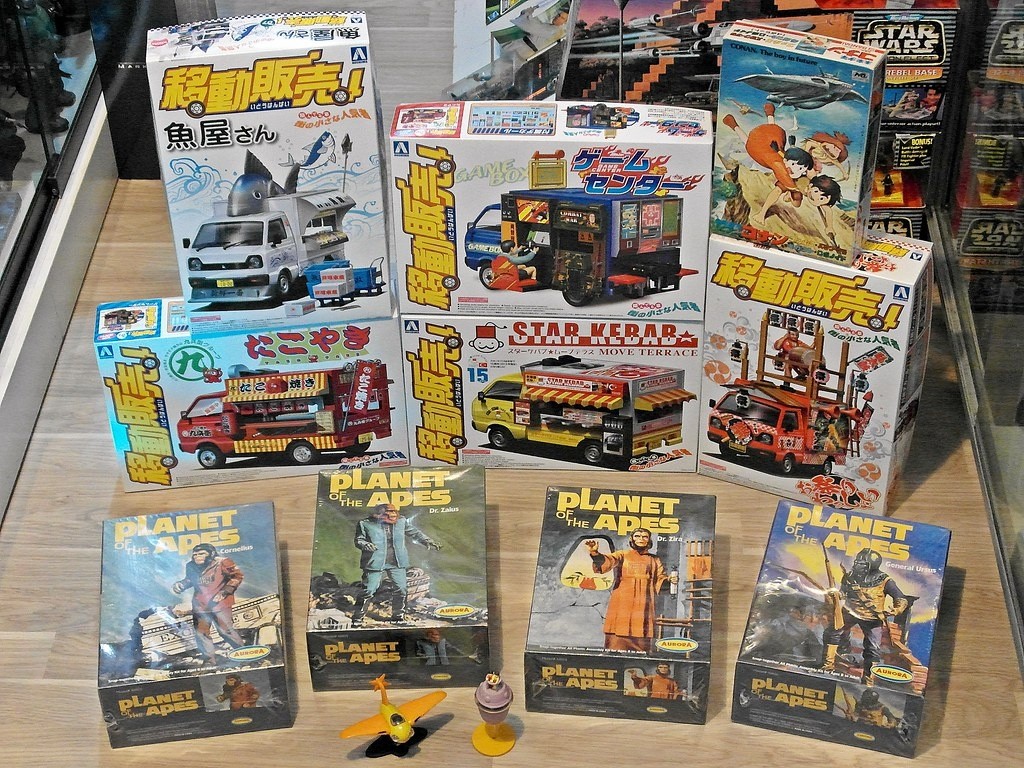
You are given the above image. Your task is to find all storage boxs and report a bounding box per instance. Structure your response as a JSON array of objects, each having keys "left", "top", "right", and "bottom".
[
  {"left": 731, "top": 500, "right": 952, "bottom": 760},
  {"left": 399, "top": 314, "right": 702, "bottom": 472},
  {"left": 524, "top": 485, "right": 716, "bottom": 726},
  {"left": 697, "top": 229, "right": 935, "bottom": 515},
  {"left": 306, "top": 463, "right": 490, "bottom": 692},
  {"left": 959, "top": 0, "right": 1024, "bottom": 315},
  {"left": 95, "top": 500, "right": 294, "bottom": 750},
  {"left": 143, "top": 9, "right": 396, "bottom": 337},
  {"left": 451, "top": 0, "right": 960, "bottom": 239},
  {"left": 710, "top": 18, "right": 891, "bottom": 268},
  {"left": 388, "top": 99, "right": 714, "bottom": 320},
  {"left": 90, "top": 298, "right": 411, "bottom": 493}
]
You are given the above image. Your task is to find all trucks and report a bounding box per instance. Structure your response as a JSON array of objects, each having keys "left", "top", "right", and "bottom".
[
  {"left": 182, "top": 185, "right": 355, "bottom": 306},
  {"left": 712, "top": 318, "right": 874, "bottom": 478},
  {"left": 471, "top": 352, "right": 698, "bottom": 466},
  {"left": 175, "top": 360, "right": 391, "bottom": 469},
  {"left": 465, "top": 186, "right": 698, "bottom": 305}
]
